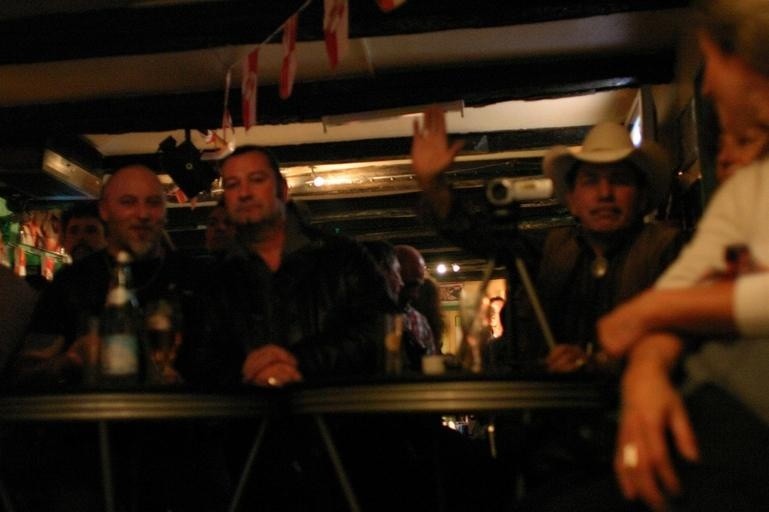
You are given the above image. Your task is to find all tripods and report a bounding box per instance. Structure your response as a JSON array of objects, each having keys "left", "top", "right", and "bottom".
[{"left": 453, "top": 231, "right": 557, "bottom": 363}]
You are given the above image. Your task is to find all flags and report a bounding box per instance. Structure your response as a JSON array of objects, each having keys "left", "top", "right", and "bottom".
[
  {"left": 239, "top": 48, "right": 258, "bottom": 132},
  {"left": 277, "top": 16, "right": 300, "bottom": 100},
  {"left": 221, "top": 61, "right": 234, "bottom": 152},
  {"left": 322, "top": 1, "right": 352, "bottom": 76}
]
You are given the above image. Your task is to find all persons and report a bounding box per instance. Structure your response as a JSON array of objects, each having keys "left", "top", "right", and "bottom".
[
  {"left": 589, "top": 1, "right": 767, "bottom": 508},
  {"left": 1, "top": 98, "right": 692, "bottom": 510},
  {"left": 712, "top": 119, "right": 767, "bottom": 187}
]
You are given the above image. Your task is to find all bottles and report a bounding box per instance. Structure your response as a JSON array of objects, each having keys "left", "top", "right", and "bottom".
[{"left": 101, "top": 265, "right": 142, "bottom": 390}]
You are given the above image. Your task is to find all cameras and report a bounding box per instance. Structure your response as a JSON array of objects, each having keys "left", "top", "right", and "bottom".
[{"left": 484, "top": 175, "right": 557, "bottom": 220}]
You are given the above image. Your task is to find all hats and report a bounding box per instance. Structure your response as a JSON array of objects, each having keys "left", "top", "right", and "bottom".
[{"left": 540, "top": 116, "right": 675, "bottom": 188}]
